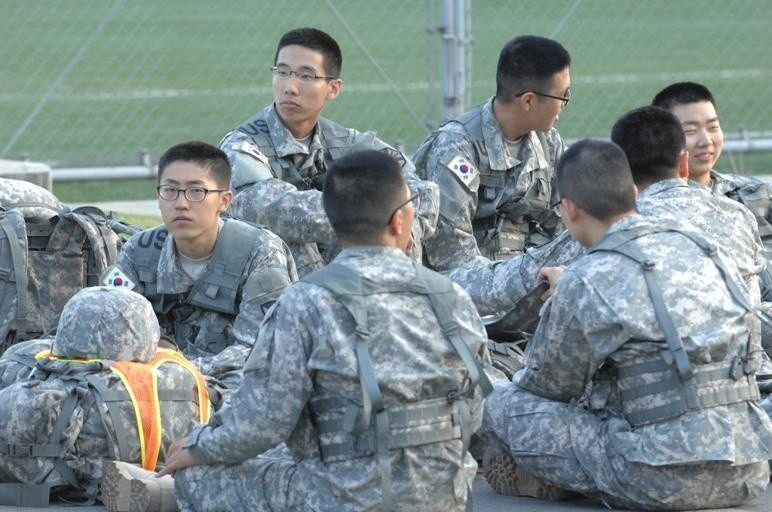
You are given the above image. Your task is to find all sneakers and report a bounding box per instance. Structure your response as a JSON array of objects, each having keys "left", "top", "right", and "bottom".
[
  {"left": 100, "top": 458, "right": 176, "bottom": 511},
  {"left": 482, "top": 444, "right": 574, "bottom": 502}
]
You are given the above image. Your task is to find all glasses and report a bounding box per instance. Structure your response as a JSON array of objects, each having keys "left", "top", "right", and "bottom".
[
  {"left": 155, "top": 185, "right": 226, "bottom": 202},
  {"left": 551, "top": 200, "right": 562, "bottom": 216},
  {"left": 387, "top": 191, "right": 419, "bottom": 226},
  {"left": 515, "top": 90, "right": 571, "bottom": 107},
  {"left": 271, "top": 67, "right": 337, "bottom": 84}
]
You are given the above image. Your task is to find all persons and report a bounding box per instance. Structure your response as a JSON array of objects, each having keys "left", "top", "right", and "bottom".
[
  {"left": 476, "top": 138, "right": 772, "bottom": 510},
  {"left": 104, "top": 140, "right": 298, "bottom": 410},
  {"left": 97, "top": 151, "right": 491, "bottom": 512},
  {"left": 651, "top": 83, "right": 772, "bottom": 359},
  {"left": 612, "top": 107, "right": 772, "bottom": 481},
  {"left": 411, "top": 35, "right": 572, "bottom": 269},
  {"left": 215, "top": 29, "right": 439, "bottom": 277}
]
